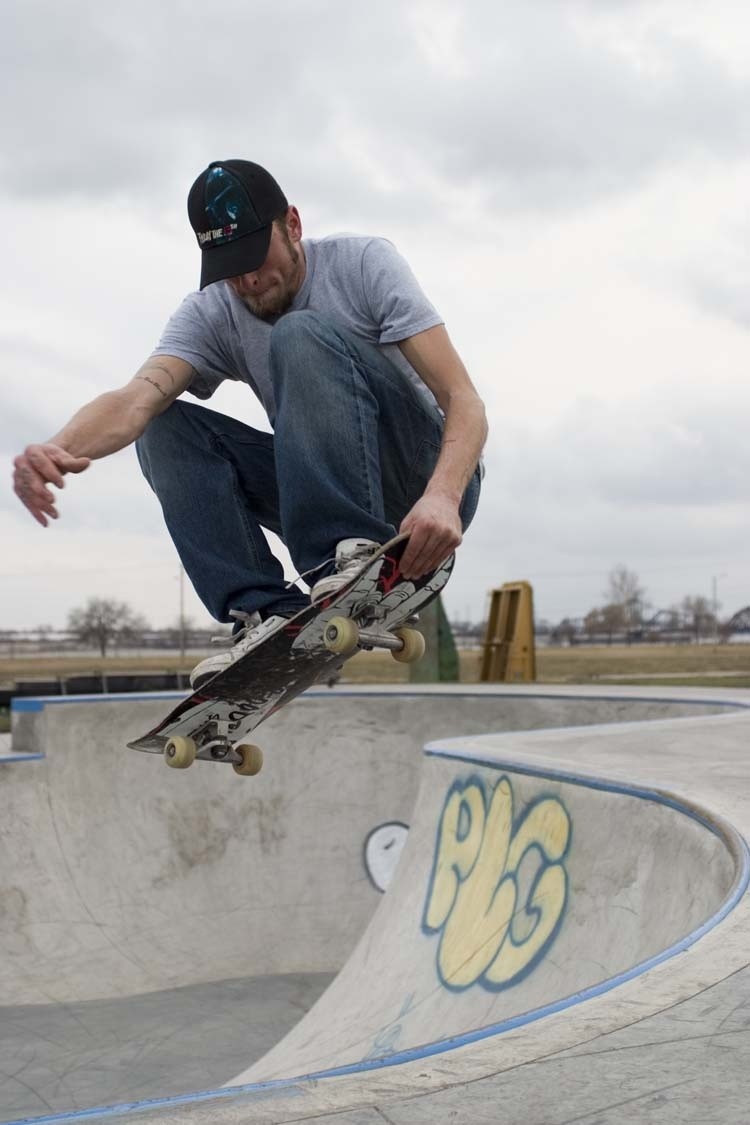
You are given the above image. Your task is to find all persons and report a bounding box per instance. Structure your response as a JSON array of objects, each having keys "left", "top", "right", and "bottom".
[{"left": 13, "top": 162, "right": 487, "bottom": 688}]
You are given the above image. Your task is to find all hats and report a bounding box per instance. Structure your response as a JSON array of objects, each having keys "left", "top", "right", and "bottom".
[{"left": 187, "top": 159, "right": 288, "bottom": 290}]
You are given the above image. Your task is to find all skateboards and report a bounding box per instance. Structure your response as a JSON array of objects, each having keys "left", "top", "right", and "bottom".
[{"left": 126, "top": 530, "right": 456, "bottom": 777}]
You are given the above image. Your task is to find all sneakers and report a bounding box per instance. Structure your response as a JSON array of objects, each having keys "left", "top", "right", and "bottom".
[
  {"left": 286, "top": 539, "right": 383, "bottom": 604},
  {"left": 188, "top": 609, "right": 290, "bottom": 690}
]
